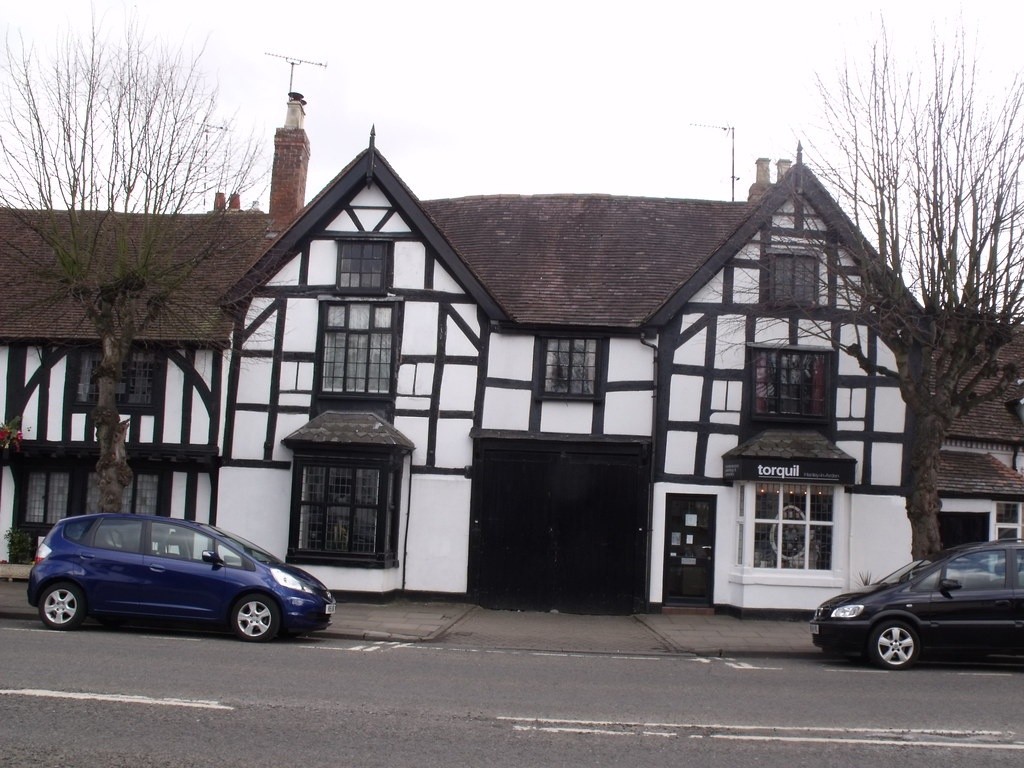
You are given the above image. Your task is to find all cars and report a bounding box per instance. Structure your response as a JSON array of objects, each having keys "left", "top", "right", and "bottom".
[{"left": 27, "top": 514, "right": 335, "bottom": 644}]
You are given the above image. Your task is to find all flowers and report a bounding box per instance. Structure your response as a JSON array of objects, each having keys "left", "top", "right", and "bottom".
[{"left": 0, "top": 416, "right": 25, "bottom": 453}]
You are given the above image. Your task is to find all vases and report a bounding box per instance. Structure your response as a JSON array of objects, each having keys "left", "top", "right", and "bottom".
[{"left": 1, "top": 448, "right": 9, "bottom": 459}]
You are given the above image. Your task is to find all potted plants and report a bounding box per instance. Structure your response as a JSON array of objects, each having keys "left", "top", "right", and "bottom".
[{"left": 0, "top": 527, "right": 38, "bottom": 582}]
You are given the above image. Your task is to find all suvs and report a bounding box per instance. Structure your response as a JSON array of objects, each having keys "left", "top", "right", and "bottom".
[{"left": 809, "top": 538, "right": 1024, "bottom": 670}]
